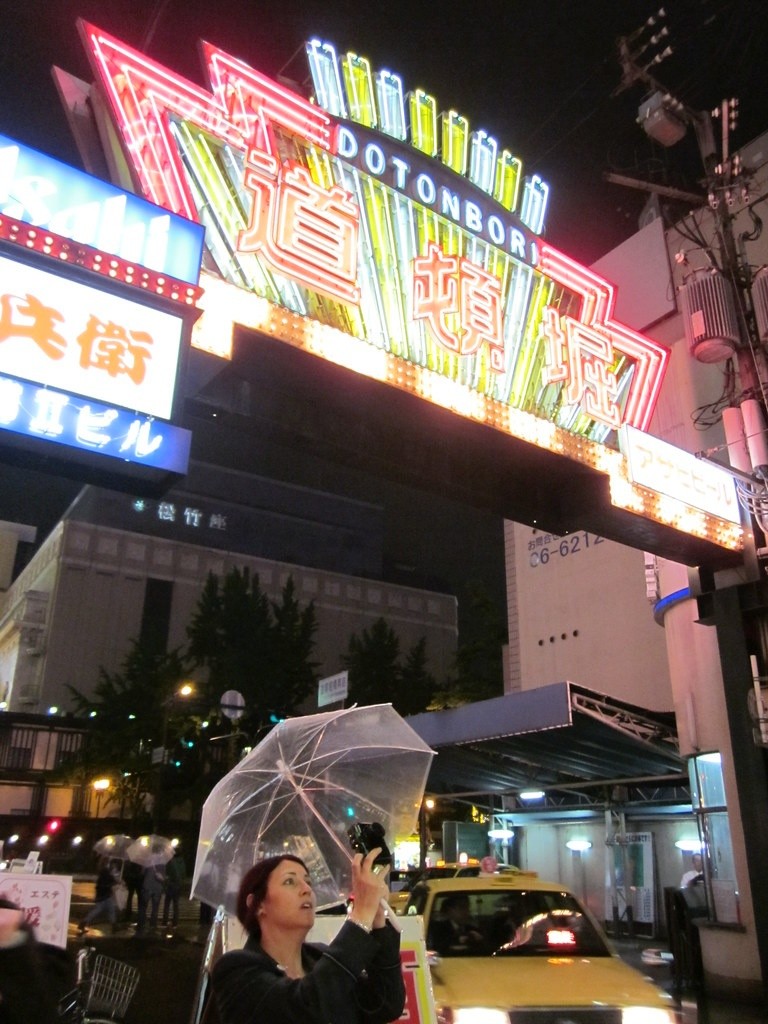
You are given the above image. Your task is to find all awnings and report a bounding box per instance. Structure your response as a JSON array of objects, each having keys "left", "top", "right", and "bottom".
[{"left": 401, "top": 679, "right": 692, "bottom": 814}]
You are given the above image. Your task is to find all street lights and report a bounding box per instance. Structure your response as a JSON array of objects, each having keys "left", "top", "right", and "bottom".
[{"left": 83, "top": 778, "right": 109, "bottom": 828}]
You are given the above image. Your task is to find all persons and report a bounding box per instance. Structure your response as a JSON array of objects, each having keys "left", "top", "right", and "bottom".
[
  {"left": 0, "top": 857, "right": 183, "bottom": 1024},
  {"left": 430, "top": 895, "right": 496, "bottom": 955},
  {"left": 680, "top": 854, "right": 703, "bottom": 889},
  {"left": 210, "top": 847, "right": 407, "bottom": 1024}
]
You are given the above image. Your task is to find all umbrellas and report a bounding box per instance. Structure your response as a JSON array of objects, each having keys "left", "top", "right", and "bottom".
[
  {"left": 188, "top": 702, "right": 439, "bottom": 934},
  {"left": 94, "top": 834, "right": 176, "bottom": 883}
]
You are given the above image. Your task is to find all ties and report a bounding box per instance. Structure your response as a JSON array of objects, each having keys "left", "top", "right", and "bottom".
[{"left": 458, "top": 923, "right": 466, "bottom": 933}]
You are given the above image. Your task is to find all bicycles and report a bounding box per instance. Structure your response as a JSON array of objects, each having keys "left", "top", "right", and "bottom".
[{"left": 46, "top": 942, "right": 141, "bottom": 1024}]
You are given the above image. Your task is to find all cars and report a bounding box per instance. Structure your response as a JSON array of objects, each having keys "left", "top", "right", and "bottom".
[{"left": 381, "top": 854, "right": 679, "bottom": 1023}]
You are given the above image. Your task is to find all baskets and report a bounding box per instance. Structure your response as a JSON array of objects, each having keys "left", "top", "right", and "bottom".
[{"left": 86, "top": 953, "right": 140, "bottom": 1018}]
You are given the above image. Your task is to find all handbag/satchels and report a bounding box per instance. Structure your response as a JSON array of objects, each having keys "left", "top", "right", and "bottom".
[{"left": 155, "top": 872, "right": 165, "bottom": 883}]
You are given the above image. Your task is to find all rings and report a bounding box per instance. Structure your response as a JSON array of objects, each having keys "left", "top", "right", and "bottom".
[{"left": 373, "top": 867, "right": 380, "bottom": 874}]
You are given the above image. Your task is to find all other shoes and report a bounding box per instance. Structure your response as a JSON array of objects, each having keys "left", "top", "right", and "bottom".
[
  {"left": 111, "top": 923, "right": 120, "bottom": 931},
  {"left": 148, "top": 926, "right": 155, "bottom": 930},
  {"left": 78, "top": 923, "right": 87, "bottom": 931},
  {"left": 134, "top": 926, "right": 143, "bottom": 930}
]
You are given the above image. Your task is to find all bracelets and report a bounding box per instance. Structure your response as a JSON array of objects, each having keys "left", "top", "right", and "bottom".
[{"left": 344, "top": 915, "right": 372, "bottom": 934}]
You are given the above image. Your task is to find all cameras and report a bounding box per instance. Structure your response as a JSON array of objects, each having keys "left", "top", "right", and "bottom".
[{"left": 347, "top": 822, "right": 392, "bottom": 867}]
